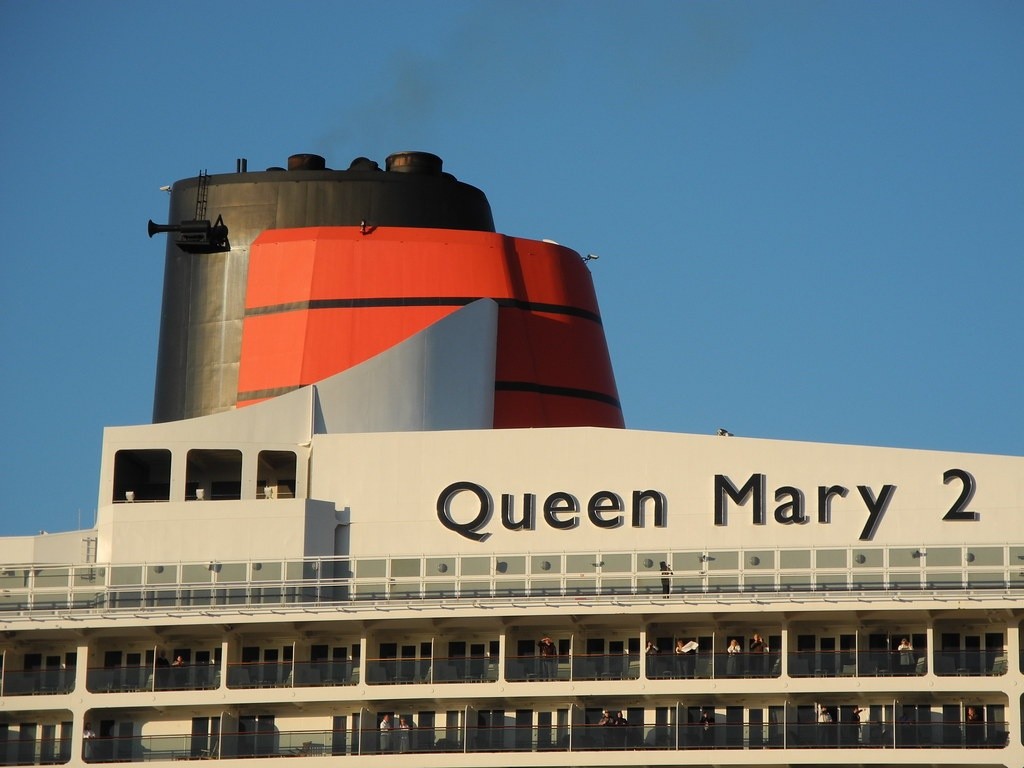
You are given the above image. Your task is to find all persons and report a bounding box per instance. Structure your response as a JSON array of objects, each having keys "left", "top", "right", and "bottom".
[
  {"left": 645, "top": 640, "right": 660, "bottom": 680},
  {"left": 675, "top": 640, "right": 688, "bottom": 680},
  {"left": 537, "top": 636, "right": 557, "bottom": 682},
  {"left": 398, "top": 717, "right": 412, "bottom": 753},
  {"left": 598, "top": 709, "right": 629, "bottom": 751},
  {"left": 898, "top": 639, "right": 915, "bottom": 676},
  {"left": 726, "top": 639, "right": 741, "bottom": 679},
  {"left": 379, "top": 714, "right": 392, "bottom": 753},
  {"left": 750, "top": 633, "right": 767, "bottom": 678},
  {"left": 698, "top": 711, "right": 715, "bottom": 750},
  {"left": 965, "top": 706, "right": 981, "bottom": 749},
  {"left": 82, "top": 722, "right": 97, "bottom": 761},
  {"left": 848, "top": 708, "right": 864, "bottom": 748},
  {"left": 816, "top": 706, "right": 833, "bottom": 749},
  {"left": 153, "top": 650, "right": 190, "bottom": 691}
]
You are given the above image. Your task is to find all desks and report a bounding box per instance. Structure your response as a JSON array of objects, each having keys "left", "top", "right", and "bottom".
[
  {"left": 41, "top": 686, "right": 60, "bottom": 695},
  {"left": 600, "top": 672, "right": 616, "bottom": 680},
  {"left": 461, "top": 675, "right": 479, "bottom": 683},
  {"left": 879, "top": 670, "right": 889, "bottom": 676},
  {"left": 525, "top": 672, "right": 547, "bottom": 682},
  {"left": 393, "top": 676, "right": 412, "bottom": 685},
  {"left": 185, "top": 681, "right": 213, "bottom": 690},
  {"left": 662, "top": 671, "right": 680, "bottom": 679},
  {"left": 257, "top": 681, "right": 274, "bottom": 688},
  {"left": 321, "top": 679, "right": 339, "bottom": 686},
  {"left": 121, "top": 684, "right": 138, "bottom": 692},
  {"left": 311, "top": 744, "right": 324, "bottom": 756},
  {"left": 956, "top": 668, "right": 970, "bottom": 676},
  {"left": 814, "top": 669, "right": 829, "bottom": 677}
]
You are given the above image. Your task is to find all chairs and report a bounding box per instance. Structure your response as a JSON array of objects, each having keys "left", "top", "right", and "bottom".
[
  {"left": 550, "top": 664, "right": 571, "bottom": 681},
  {"left": 984, "top": 656, "right": 1007, "bottom": 676},
  {"left": 275, "top": 670, "right": 292, "bottom": 687},
  {"left": 621, "top": 661, "right": 640, "bottom": 679},
  {"left": 579, "top": 736, "right": 602, "bottom": 747},
  {"left": 62, "top": 681, "right": 75, "bottom": 695},
  {"left": 837, "top": 665, "right": 856, "bottom": 677},
  {"left": 239, "top": 669, "right": 257, "bottom": 688},
  {"left": 413, "top": 666, "right": 431, "bottom": 684},
  {"left": 137, "top": 674, "right": 153, "bottom": 692},
  {"left": 200, "top": 739, "right": 219, "bottom": 760},
  {"left": 479, "top": 664, "right": 498, "bottom": 683},
  {"left": 683, "top": 734, "right": 704, "bottom": 750},
  {"left": 342, "top": 667, "right": 359, "bottom": 686},
  {"left": 31, "top": 683, "right": 40, "bottom": 695},
  {"left": 913, "top": 657, "right": 927, "bottom": 676},
  {"left": 288, "top": 741, "right": 312, "bottom": 757},
  {"left": 789, "top": 732, "right": 816, "bottom": 749},
  {"left": 984, "top": 730, "right": 1009, "bottom": 748}
]
